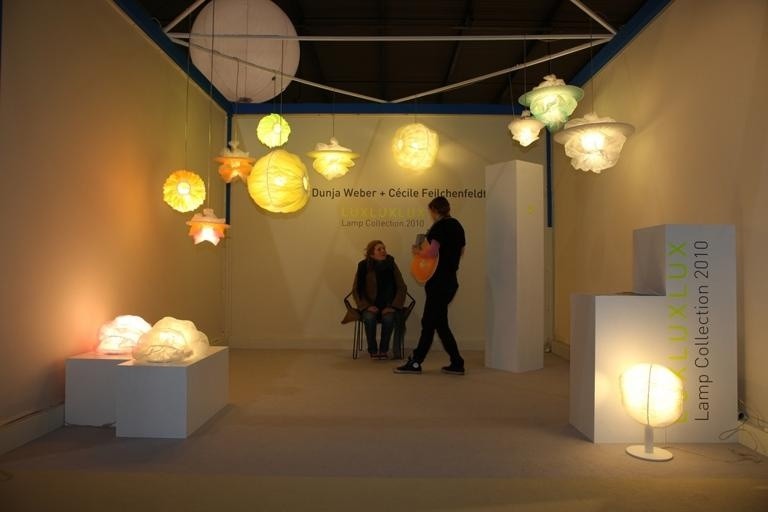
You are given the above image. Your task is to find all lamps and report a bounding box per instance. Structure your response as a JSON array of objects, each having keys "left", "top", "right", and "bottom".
[
  {"left": 126, "top": 313, "right": 212, "bottom": 370},
  {"left": 93, "top": 310, "right": 152, "bottom": 358},
  {"left": 620, "top": 357, "right": 688, "bottom": 464}
]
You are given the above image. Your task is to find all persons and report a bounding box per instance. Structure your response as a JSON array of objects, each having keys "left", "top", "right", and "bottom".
[
  {"left": 352, "top": 239, "right": 408, "bottom": 361},
  {"left": 392, "top": 195, "right": 466, "bottom": 375}
]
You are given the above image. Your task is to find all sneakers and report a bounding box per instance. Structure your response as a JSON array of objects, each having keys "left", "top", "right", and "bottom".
[
  {"left": 440, "top": 356, "right": 465, "bottom": 375},
  {"left": 369, "top": 350, "right": 388, "bottom": 361},
  {"left": 395, "top": 355, "right": 424, "bottom": 373}
]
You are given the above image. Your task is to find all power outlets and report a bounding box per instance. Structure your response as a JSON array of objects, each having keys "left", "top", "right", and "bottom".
[{"left": 736, "top": 399, "right": 747, "bottom": 421}]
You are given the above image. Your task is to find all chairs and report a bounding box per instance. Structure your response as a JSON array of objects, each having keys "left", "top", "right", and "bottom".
[{"left": 343, "top": 289, "right": 416, "bottom": 361}]
